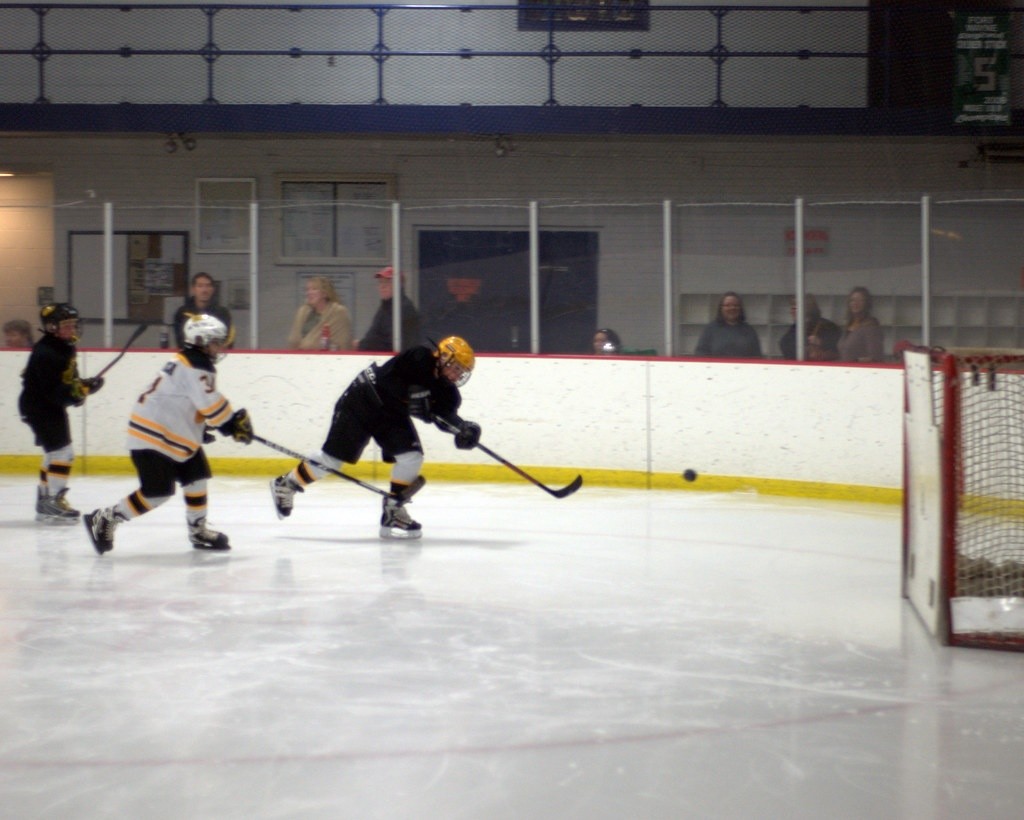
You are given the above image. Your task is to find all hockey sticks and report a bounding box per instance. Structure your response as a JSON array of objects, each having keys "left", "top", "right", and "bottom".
[
  {"left": 96, "top": 322, "right": 149, "bottom": 379},
  {"left": 433, "top": 415, "right": 583, "bottom": 499},
  {"left": 250, "top": 433, "right": 426, "bottom": 502}
]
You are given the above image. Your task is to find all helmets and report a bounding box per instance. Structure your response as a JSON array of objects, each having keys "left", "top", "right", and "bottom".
[
  {"left": 183, "top": 314, "right": 229, "bottom": 365},
  {"left": 37, "top": 303, "right": 83, "bottom": 347},
  {"left": 426, "top": 336, "right": 476, "bottom": 387}
]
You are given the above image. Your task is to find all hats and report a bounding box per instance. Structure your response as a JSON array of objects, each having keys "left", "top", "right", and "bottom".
[
  {"left": 374, "top": 267, "right": 404, "bottom": 280},
  {"left": 598, "top": 329, "right": 620, "bottom": 345}
]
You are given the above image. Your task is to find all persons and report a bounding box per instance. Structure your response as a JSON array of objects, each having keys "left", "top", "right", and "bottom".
[
  {"left": 893, "top": 340, "right": 914, "bottom": 362},
  {"left": 287, "top": 276, "right": 353, "bottom": 349},
  {"left": 18, "top": 303, "right": 106, "bottom": 517},
  {"left": 92, "top": 314, "right": 254, "bottom": 551},
  {"left": 173, "top": 272, "right": 236, "bottom": 350},
  {"left": 274, "top": 335, "right": 481, "bottom": 530},
  {"left": 807, "top": 319, "right": 841, "bottom": 360},
  {"left": 694, "top": 293, "right": 761, "bottom": 357},
  {"left": 779, "top": 293, "right": 822, "bottom": 360},
  {"left": 353, "top": 266, "right": 419, "bottom": 351},
  {"left": 3, "top": 319, "right": 34, "bottom": 347},
  {"left": 592, "top": 329, "right": 620, "bottom": 354},
  {"left": 837, "top": 287, "right": 884, "bottom": 362}
]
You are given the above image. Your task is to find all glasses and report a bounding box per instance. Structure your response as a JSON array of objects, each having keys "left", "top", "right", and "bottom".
[{"left": 721, "top": 303, "right": 740, "bottom": 309}]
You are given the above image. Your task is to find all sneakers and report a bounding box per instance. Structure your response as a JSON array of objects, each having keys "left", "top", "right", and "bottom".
[
  {"left": 35, "top": 485, "right": 81, "bottom": 525},
  {"left": 269, "top": 472, "right": 305, "bottom": 521},
  {"left": 83, "top": 507, "right": 130, "bottom": 556},
  {"left": 186, "top": 516, "right": 231, "bottom": 550},
  {"left": 379, "top": 506, "right": 423, "bottom": 539}
]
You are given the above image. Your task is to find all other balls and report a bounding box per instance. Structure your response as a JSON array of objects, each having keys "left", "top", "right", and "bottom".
[{"left": 684, "top": 469, "right": 696, "bottom": 482}]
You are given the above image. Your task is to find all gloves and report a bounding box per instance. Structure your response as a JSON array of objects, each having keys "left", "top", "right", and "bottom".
[
  {"left": 203, "top": 426, "right": 216, "bottom": 444},
  {"left": 233, "top": 408, "right": 253, "bottom": 445},
  {"left": 409, "top": 386, "right": 434, "bottom": 423},
  {"left": 72, "top": 377, "right": 104, "bottom": 407},
  {"left": 455, "top": 421, "right": 482, "bottom": 449}
]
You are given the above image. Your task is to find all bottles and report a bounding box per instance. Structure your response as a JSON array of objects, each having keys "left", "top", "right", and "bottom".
[{"left": 320, "top": 323, "right": 330, "bottom": 351}]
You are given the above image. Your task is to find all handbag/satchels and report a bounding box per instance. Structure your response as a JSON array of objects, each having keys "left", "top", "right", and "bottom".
[{"left": 807, "top": 320, "right": 835, "bottom": 361}]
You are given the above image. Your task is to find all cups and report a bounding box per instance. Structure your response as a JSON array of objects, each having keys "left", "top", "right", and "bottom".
[{"left": 160, "top": 333, "right": 169, "bottom": 349}]
